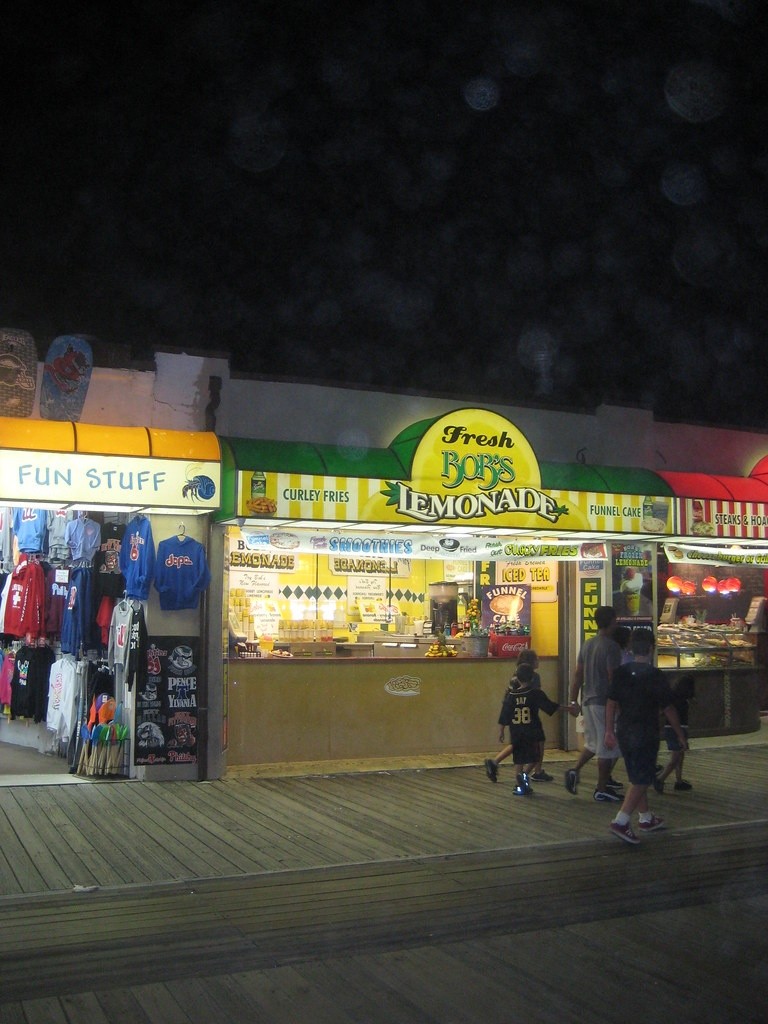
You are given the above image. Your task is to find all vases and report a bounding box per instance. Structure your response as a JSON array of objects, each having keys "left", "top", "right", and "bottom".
[{"left": 460, "top": 635, "right": 491, "bottom": 657}]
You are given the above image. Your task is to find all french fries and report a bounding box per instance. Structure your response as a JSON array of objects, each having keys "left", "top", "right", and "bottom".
[{"left": 247, "top": 497, "right": 277, "bottom": 512}]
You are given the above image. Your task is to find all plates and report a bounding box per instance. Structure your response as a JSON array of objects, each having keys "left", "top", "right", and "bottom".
[
  {"left": 642, "top": 518, "right": 666, "bottom": 533},
  {"left": 270, "top": 533, "right": 299, "bottom": 549},
  {"left": 489, "top": 595, "right": 524, "bottom": 615},
  {"left": 271, "top": 650, "right": 293, "bottom": 657}
]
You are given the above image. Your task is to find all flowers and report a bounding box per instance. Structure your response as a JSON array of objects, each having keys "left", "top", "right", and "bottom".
[
  {"left": 464, "top": 597, "right": 484, "bottom": 634},
  {"left": 426, "top": 630, "right": 459, "bottom": 658}
]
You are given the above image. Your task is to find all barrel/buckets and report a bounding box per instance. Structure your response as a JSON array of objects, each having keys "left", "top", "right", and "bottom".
[
  {"left": 259, "top": 639, "right": 273, "bottom": 653},
  {"left": 464, "top": 634, "right": 490, "bottom": 657}
]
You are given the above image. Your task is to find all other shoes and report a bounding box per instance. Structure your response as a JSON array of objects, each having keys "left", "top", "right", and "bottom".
[
  {"left": 653, "top": 765, "right": 664, "bottom": 776},
  {"left": 674, "top": 781, "right": 692, "bottom": 791},
  {"left": 654, "top": 777, "right": 665, "bottom": 795},
  {"left": 512, "top": 785, "right": 533, "bottom": 795},
  {"left": 517, "top": 771, "right": 531, "bottom": 795}
]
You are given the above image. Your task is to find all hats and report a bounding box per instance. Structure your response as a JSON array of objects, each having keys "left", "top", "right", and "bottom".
[{"left": 674, "top": 675, "right": 698, "bottom": 705}]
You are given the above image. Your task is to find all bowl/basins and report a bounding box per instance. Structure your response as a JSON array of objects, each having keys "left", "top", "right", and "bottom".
[
  {"left": 250, "top": 505, "right": 277, "bottom": 517},
  {"left": 690, "top": 522, "right": 713, "bottom": 537},
  {"left": 685, "top": 658, "right": 698, "bottom": 664}
]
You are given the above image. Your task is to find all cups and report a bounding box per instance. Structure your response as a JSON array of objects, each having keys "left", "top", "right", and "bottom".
[
  {"left": 653, "top": 501, "right": 668, "bottom": 524},
  {"left": 576, "top": 716, "right": 585, "bottom": 732},
  {"left": 732, "top": 618, "right": 740, "bottom": 626},
  {"left": 278, "top": 620, "right": 333, "bottom": 642},
  {"left": 229, "top": 587, "right": 254, "bottom": 641},
  {"left": 685, "top": 617, "right": 695, "bottom": 625},
  {"left": 396, "top": 616, "right": 427, "bottom": 636},
  {"left": 621, "top": 573, "right": 642, "bottom": 616}
]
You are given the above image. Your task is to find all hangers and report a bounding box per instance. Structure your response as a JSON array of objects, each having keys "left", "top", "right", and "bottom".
[{"left": 0, "top": 505, "right": 206, "bottom": 687}]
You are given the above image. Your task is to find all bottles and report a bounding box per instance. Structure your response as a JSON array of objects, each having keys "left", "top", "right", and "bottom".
[
  {"left": 495, "top": 621, "right": 530, "bottom": 636},
  {"left": 643, "top": 495, "right": 653, "bottom": 520},
  {"left": 693, "top": 501, "right": 703, "bottom": 523},
  {"left": 465, "top": 617, "right": 490, "bottom": 636},
  {"left": 435, "top": 624, "right": 442, "bottom": 637},
  {"left": 444, "top": 623, "right": 450, "bottom": 637},
  {"left": 251, "top": 471, "right": 266, "bottom": 499},
  {"left": 451, "top": 624, "right": 457, "bottom": 637}
]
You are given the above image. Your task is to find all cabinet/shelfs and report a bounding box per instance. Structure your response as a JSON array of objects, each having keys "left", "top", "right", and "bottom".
[
  {"left": 656, "top": 625, "right": 766, "bottom": 742},
  {"left": 373, "top": 635, "right": 459, "bottom": 656}
]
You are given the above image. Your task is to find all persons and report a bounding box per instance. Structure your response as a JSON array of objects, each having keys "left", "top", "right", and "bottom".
[
  {"left": 566, "top": 607, "right": 625, "bottom": 801},
  {"left": 655, "top": 675, "right": 696, "bottom": 794},
  {"left": 499, "top": 663, "right": 578, "bottom": 795},
  {"left": 597, "top": 627, "right": 634, "bottom": 789},
  {"left": 604, "top": 629, "right": 665, "bottom": 844},
  {"left": 484, "top": 651, "right": 555, "bottom": 781}
]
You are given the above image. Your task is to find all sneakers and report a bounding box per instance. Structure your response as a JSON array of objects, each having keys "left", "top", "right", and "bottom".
[
  {"left": 531, "top": 769, "right": 553, "bottom": 782},
  {"left": 608, "top": 819, "right": 640, "bottom": 844},
  {"left": 606, "top": 775, "right": 623, "bottom": 789},
  {"left": 637, "top": 812, "right": 665, "bottom": 832},
  {"left": 485, "top": 759, "right": 499, "bottom": 783},
  {"left": 593, "top": 788, "right": 625, "bottom": 801},
  {"left": 565, "top": 769, "right": 577, "bottom": 794}
]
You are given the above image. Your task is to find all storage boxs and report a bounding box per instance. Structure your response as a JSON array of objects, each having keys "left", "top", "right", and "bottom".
[{"left": 489, "top": 629, "right": 531, "bottom": 658}]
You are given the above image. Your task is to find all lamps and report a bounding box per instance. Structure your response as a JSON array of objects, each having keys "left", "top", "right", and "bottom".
[{"left": 668, "top": 562, "right": 740, "bottom": 595}]
